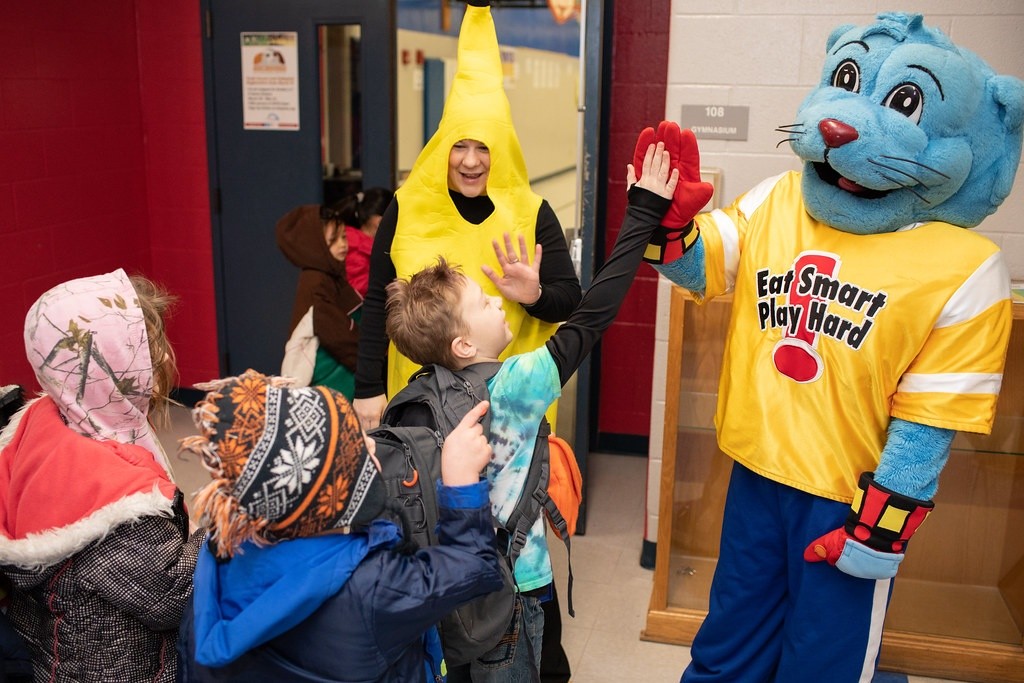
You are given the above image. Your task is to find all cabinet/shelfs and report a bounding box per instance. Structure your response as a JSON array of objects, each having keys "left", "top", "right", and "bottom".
[{"left": 637, "top": 280, "right": 1022, "bottom": 683}]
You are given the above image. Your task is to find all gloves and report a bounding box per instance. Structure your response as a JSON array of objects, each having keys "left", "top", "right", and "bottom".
[
  {"left": 634, "top": 121, "right": 712, "bottom": 264},
  {"left": 803, "top": 469, "right": 934, "bottom": 580}
]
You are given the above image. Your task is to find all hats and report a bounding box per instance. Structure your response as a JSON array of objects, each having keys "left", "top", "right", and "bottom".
[{"left": 176, "top": 369, "right": 412, "bottom": 563}]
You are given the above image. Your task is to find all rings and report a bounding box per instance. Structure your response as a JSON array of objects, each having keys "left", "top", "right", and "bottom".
[{"left": 509, "top": 258, "right": 519, "bottom": 264}]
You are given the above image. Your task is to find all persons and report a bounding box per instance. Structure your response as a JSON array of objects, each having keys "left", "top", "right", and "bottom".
[
  {"left": 0, "top": 266, "right": 210, "bottom": 682},
  {"left": 333, "top": 187, "right": 395, "bottom": 300},
  {"left": 173, "top": 368, "right": 504, "bottom": 682},
  {"left": 624, "top": 10, "right": 1024, "bottom": 682},
  {"left": 352, "top": 124, "right": 582, "bottom": 436},
  {"left": 383, "top": 140, "right": 679, "bottom": 681},
  {"left": 275, "top": 202, "right": 360, "bottom": 406}
]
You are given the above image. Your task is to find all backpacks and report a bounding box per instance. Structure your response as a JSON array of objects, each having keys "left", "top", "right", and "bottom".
[{"left": 361, "top": 363, "right": 576, "bottom": 669}]
